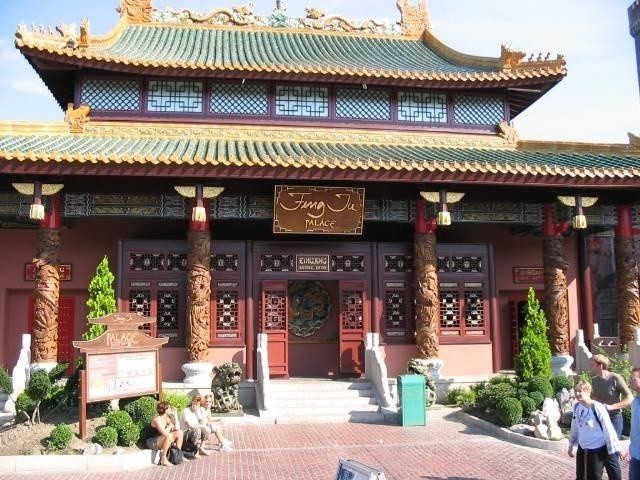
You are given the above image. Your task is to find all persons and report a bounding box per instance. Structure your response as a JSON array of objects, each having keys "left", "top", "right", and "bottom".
[
  {"left": 588, "top": 354, "right": 634, "bottom": 441},
  {"left": 619, "top": 365, "right": 640, "bottom": 480},
  {"left": 200, "top": 394, "right": 233, "bottom": 452},
  {"left": 146, "top": 401, "right": 190, "bottom": 467},
  {"left": 180, "top": 396, "right": 211, "bottom": 459},
  {"left": 566, "top": 379, "right": 628, "bottom": 480}
]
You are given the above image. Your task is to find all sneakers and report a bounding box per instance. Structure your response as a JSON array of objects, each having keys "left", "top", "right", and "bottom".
[
  {"left": 219, "top": 445, "right": 232, "bottom": 452},
  {"left": 218, "top": 440, "right": 232, "bottom": 447}
]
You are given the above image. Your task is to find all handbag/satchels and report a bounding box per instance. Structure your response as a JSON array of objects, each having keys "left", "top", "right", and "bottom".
[
  {"left": 167, "top": 446, "right": 184, "bottom": 465},
  {"left": 183, "top": 430, "right": 198, "bottom": 458}
]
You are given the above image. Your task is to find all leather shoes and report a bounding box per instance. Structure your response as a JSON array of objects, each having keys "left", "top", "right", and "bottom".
[
  {"left": 200, "top": 447, "right": 210, "bottom": 456},
  {"left": 161, "top": 459, "right": 173, "bottom": 467}
]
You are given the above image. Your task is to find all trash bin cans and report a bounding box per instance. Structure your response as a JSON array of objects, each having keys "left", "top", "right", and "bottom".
[{"left": 397, "top": 374, "right": 426, "bottom": 427}]
